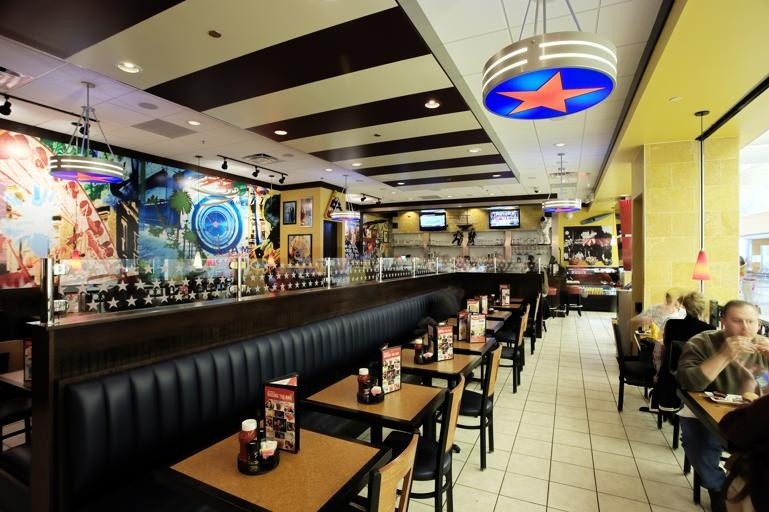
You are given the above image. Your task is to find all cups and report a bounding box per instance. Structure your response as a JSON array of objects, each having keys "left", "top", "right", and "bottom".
[
  {"left": 53, "top": 299, "right": 70, "bottom": 315},
  {"left": 641, "top": 317, "right": 650, "bottom": 330},
  {"left": 474, "top": 238, "right": 537, "bottom": 246}
]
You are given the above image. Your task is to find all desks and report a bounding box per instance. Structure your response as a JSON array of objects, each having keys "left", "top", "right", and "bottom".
[
  {"left": 304, "top": 375, "right": 446, "bottom": 449},
  {"left": 170, "top": 424, "right": 379, "bottom": 509},
  {"left": 457, "top": 289, "right": 542, "bottom": 393},
  {"left": 400, "top": 349, "right": 480, "bottom": 384}
]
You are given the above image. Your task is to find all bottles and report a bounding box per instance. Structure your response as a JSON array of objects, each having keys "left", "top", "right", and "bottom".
[
  {"left": 239, "top": 418, "right": 265, "bottom": 473},
  {"left": 491, "top": 293, "right": 500, "bottom": 306},
  {"left": 439, "top": 322, "right": 445, "bottom": 326},
  {"left": 414, "top": 338, "right": 428, "bottom": 358},
  {"left": 357, "top": 368, "right": 371, "bottom": 396}
]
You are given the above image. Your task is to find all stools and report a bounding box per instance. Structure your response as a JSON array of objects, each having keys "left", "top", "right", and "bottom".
[
  {"left": 544, "top": 288, "right": 558, "bottom": 320},
  {"left": 565, "top": 284, "right": 585, "bottom": 317}
]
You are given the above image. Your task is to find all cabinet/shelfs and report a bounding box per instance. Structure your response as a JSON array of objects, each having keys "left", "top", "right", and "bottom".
[
  {"left": 426, "top": 231, "right": 462, "bottom": 248},
  {"left": 510, "top": 229, "right": 552, "bottom": 247},
  {"left": 391, "top": 231, "right": 424, "bottom": 248},
  {"left": 468, "top": 231, "right": 507, "bottom": 249}
]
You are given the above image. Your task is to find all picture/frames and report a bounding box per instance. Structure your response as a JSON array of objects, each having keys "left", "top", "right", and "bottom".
[
  {"left": 282, "top": 200, "right": 297, "bottom": 225},
  {"left": 287, "top": 234, "right": 313, "bottom": 265},
  {"left": 299, "top": 196, "right": 313, "bottom": 228}
]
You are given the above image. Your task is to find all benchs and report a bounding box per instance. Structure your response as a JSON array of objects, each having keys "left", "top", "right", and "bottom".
[{"left": 48, "top": 287, "right": 460, "bottom": 512}]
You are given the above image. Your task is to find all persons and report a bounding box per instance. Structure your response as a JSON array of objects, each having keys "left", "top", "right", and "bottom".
[
  {"left": 266, "top": 399, "right": 294, "bottom": 449},
  {"left": 663, "top": 292, "right": 717, "bottom": 368},
  {"left": 289, "top": 203, "right": 295, "bottom": 222},
  {"left": 676, "top": 301, "right": 769, "bottom": 510},
  {"left": 394, "top": 253, "right": 537, "bottom": 273},
  {"left": 631, "top": 287, "right": 687, "bottom": 377},
  {"left": 437, "top": 333, "right": 453, "bottom": 360},
  {"left": 580, "top": 232, "right": 612, "bottom": 263},
  {"left": 383, "top": 358, "right": 400, "bottom": 390}
]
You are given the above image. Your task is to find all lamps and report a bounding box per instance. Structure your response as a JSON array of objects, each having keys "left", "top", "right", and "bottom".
[
  {"left": 482, "top": 0, "right": 619, "bottom": 121},
  {"left": 49, "top": 81, "right": 125, "bottom": 186},
  {"left": 327, "top": 174, "right": 360, "bottom": 220},
  {"left": 215, "top": 154, "right": 290, "bottom": 183},
  {"left": 693, "top": 110, "right": 712, "bottom": 282},
  {"left": 541, "top": 154, "right": 583, "bottom": 214},
  {"left": 0, "top": 92, "right": 102, "bottom": 134},
  {"left": 361, "top": 193, "right": 382, "bottom": 206}
]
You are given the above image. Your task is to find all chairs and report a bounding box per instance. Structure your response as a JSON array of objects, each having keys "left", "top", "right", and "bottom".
[
  {"left": 610, "top": 313, "right": 653, "bottom": 413},
  {"left": 438, "top": 342, "right": 501, "bottom": 469},
  {"left": 345, "top": 431, "right": 420, "bottom": 510},
  {"left": 392, "top": 373, "right": 465, "bottom": 511},
  {"left": 1, "top": 340, "right": 26, "bottom": 452}
]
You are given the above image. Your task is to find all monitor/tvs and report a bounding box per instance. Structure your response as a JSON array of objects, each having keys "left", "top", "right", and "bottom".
[
  {"left": 489, "top": 209, "right": 520, "bottom": 229},
  {"left": 419, "top": 212, "right": 446, "bottom": 231}
]
